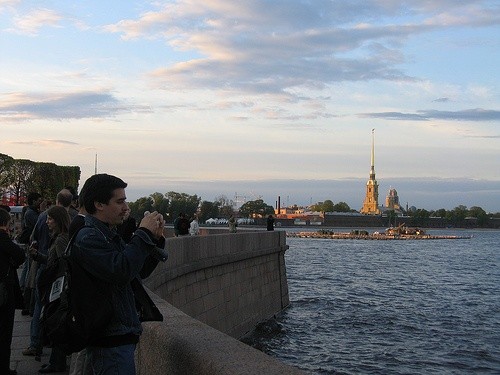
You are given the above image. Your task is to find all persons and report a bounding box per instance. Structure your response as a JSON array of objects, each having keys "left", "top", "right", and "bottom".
[
  {"left": 73, "top": 174, "right": 169, "bottom": 375},
  {"left": 174, "top": 210, "right": 200, "bottom": 237},
  {"left": 267, "top": 215, "right": 275, "bottom": 231},
  {"left": 226, "top": 214, "right": 238, "bottom": 233},
  {"left": 0, "top": 187, "right": 151, "bottom": 375}
]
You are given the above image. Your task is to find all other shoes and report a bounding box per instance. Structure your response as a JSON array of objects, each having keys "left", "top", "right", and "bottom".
[
  {"left": 21, "top": 310, "right": 32, "bottom": 315},
  {"left": 9, "top": 370, "right": 18, "bottom": 375},
  {"left": 22, "top": 347, "right": 43, "bottom": 357},
  {"left": 37, "top": 365, "right": 71, "bottom": 375}
]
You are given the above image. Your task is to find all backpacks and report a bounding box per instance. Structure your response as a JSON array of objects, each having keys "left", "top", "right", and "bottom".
[{"left": 39, "top": 225, "right": 115, "bottom": 355}]
